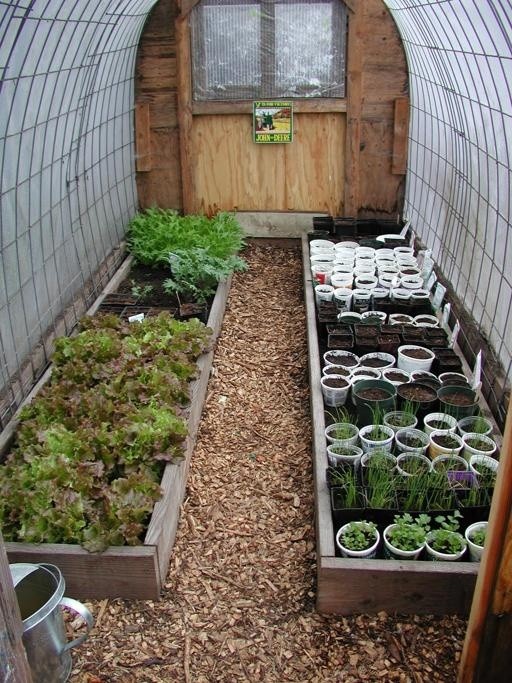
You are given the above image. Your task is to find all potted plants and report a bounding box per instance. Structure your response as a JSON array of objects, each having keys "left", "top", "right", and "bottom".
[
  {"left": 431, "top": 450, "right": 469, "bottom": 476},
  {"left": 422, "top": 392, "right": 458, "bottom": 435},
  {"left": 461, "top": 432, "right": 498, "bottom": 460},
  {"left": 361, "top": 440, "right": 398, "bottom": 474},
  {"left": 465, "top": 521, "right": 489, "bottom": 563},
  {"left": 446, "top": 469, "right": 478, "bottom": 490},
  {"left": 468, "top": 454, "right": 499, "bottom": 477},
  {"left": 480, "top": 484, "right": 494, "bottom": 509},
  {"left": 454, "top": 483, "right": 481, "bottom": 508},
  {"left": 457, "top": 407, "right": 495, "bottom": 437},
  {"left": 397, "top": 449, "right": 431, "bottom": 478},
  {"left": 327, "top": 460, "right": 359, "bottom": 487},
  {"left": 392, "top": 470, "right": 425, "bottom": 491},
  {"left": 473, "top": 471, "right": 495, "bottom": 488},
  {"left": 336, "top": 519, "right": 380, "bottom": 560},
  {"left": 357, "top": 401, "right": 395, "bottom": 454},
  {"left": 363, "top": 478, "right": 394, "bottom": 510},
  {"left": 426, "top": 509, "right": 468, "bottom": 562},
  {"left": 396, "top": 484, "right": 427, "bottom": 512},
  {"left": 323, "top": 403, "right": 360, "bottom": 446},
  {"left": 326, "top": 423, "right": 363, "bottom": 466},
  {"left": 360, "top": 462, "right": 388, "bottom": 487},
  {"left": 382, "top": 513, "right": 431, "bottom": 560},
  {"left": 383, "top": 396, "right": 421, "bottom": 432},
  {"left": 424, "top": 463, "right": 448, "bottom": 490},
  {"left": 394, "top": 428, "right": 430, "bottom": 458},
  {"left": 426, "top": 485, "right": 457, "bottom": 511},
  {"left": 428, "top": 429, "right": 462, "bottom": 460},
  {"left": 331, "top": 484, "right": 363, "bottom": 510}
]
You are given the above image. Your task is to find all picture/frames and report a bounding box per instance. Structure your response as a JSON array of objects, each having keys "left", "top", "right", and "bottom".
[{"left": 252, "top": 101, "right": 293, "bottom": 144}]
